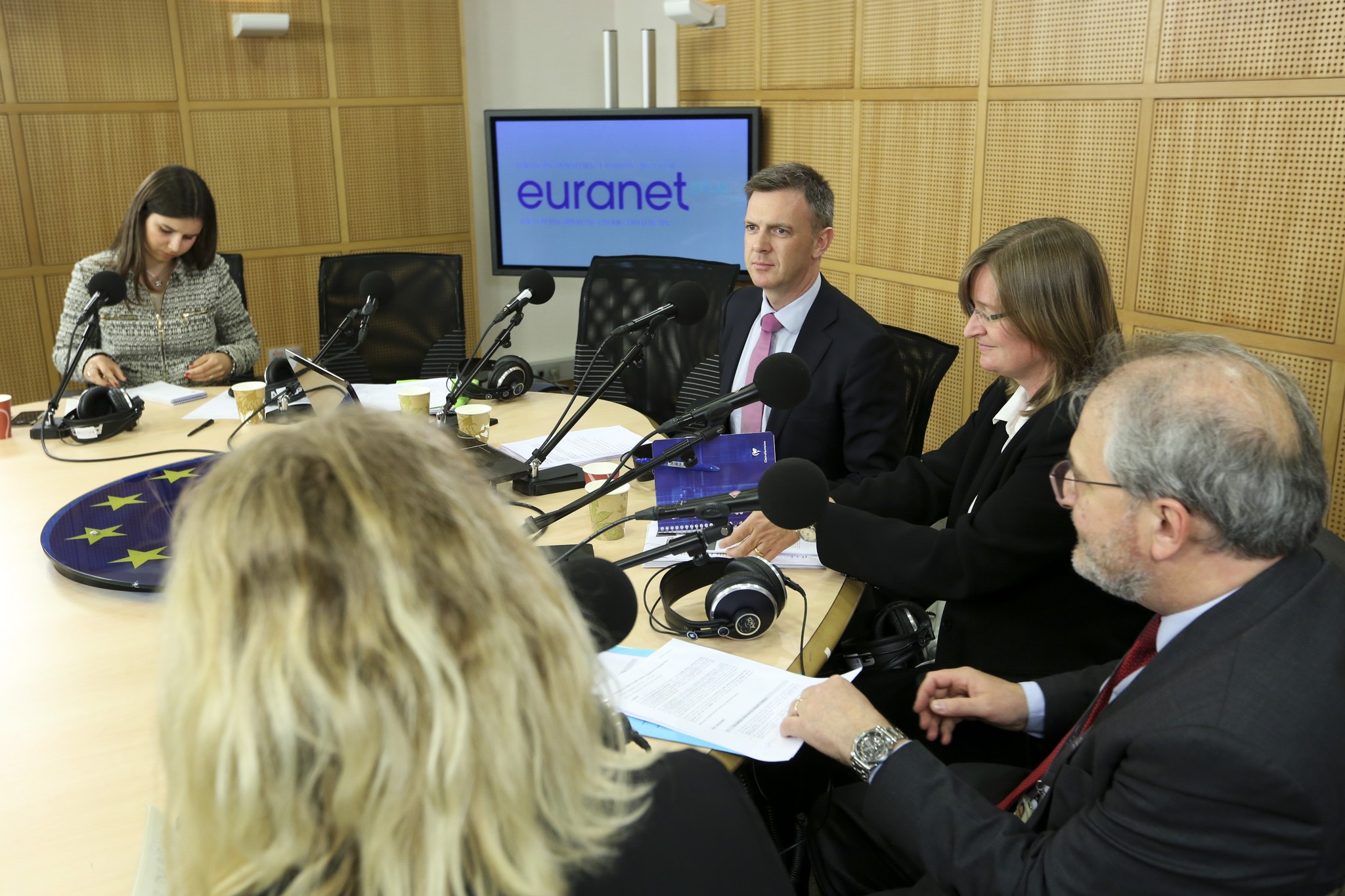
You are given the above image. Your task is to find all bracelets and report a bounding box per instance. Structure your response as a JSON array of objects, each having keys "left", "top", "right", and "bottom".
[{"left": 220, "top": 348, "right": 237, "bottom": 377}]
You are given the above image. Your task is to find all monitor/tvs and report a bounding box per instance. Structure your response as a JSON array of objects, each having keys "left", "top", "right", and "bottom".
[{"left": 487, "top": 108, "right": 765, "bottom": 286}]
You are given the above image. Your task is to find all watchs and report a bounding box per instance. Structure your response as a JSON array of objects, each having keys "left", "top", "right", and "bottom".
[
  {"left": 847, "top": 724, "right": 907, "bottom": 784},
  {"left": 793, "top": 525, "right": 818, "bottom": 543}
]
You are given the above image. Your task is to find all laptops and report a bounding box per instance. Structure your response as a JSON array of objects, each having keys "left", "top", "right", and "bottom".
[{"left": 285, "top": 347, "right": 531, "bottom": 486}]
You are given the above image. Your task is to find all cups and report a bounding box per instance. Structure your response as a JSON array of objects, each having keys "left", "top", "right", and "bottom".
[
  {"left": 0, "top": 392, "right": 13, "bottom": 440},
  {"left": 232, "top": 380, "right": 267, "bottom": 426},
  {"left": 398, "top": 385, "right": 431, "bottom": 421},
  {"left": 585, "top": 478, "right": 631, "bottom": 541},
  {"left": 582, "top": 462, "right": 620, "bottom": 486},
  {"left": 454, "top": 404, "right": 492, "bottom": 444}
]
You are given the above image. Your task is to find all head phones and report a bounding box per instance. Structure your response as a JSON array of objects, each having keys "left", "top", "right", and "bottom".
[
  {"left": 453, "top": 355, "right": 538, "bottom": 400},
  {"left": 260, "top": 356, "right": 296, "bottom": 409},
  {"left": 644, "top": 555, "right": 790, "bottom": 643},
  {"left": 842, "top": 600, "right": 936, "bottom": 675},
  {"left": 64, "top": 386, "right": 145, "bottom": 443}
]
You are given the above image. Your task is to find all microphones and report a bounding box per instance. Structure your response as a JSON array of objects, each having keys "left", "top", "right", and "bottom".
[
  {"left": 492, "top": 269, "right": 557, "bottom": 325},
  {"left": 668, "top": 350, "right": 811, "bottom": 435},
  {"left": 354, "top": 271, "right": 394, "bottom": 344},
  {"left": 75, "top": 271, "right": 128, "bottom": 326},
  {"left": 639, "top": 456, "right": 829, "bottom": 530},
  {"left": 612, "top": 280, "right": 711, "bottom": 339}
]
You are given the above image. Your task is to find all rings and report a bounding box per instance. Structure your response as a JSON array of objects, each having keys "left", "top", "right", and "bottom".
[
  {"left": 87, "top": 364, "right": 97, "bottom": 371},
  {"left": 208, "top": 373, "right": 214, "bottom": 380},
  {"left": 87, "top": 371, "right": 91, "bottom": 377},
  {"left": 754, "top": 548, "right": 765, "bottom": 557},
  {"left": 795, "top": 699, "right": 802, "bottom": 717}
]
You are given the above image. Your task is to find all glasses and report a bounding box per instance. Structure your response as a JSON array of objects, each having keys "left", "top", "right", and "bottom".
[
  {"left": 1049, "top": 460, "right": 1125, "bottom": 510},
  {"left": 964, "top": 297, "right": 1015, "bottom": 328}
]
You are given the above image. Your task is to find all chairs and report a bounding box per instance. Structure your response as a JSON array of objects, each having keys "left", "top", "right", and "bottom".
[
  {"left": 316, "top": 250, "right": 467, "bottom": 384},
  {"left": 575, "top": 252, "right": 736, "bottom": 441},
  {"left": 210, "top": 252, "right": 257, "bottom": 384},
  {"left": 878, "top": 323, "right": 962, "bottom": 466}
]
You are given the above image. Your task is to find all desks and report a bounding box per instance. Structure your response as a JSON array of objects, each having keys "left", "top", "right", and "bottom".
[{"left": 0, "top": 386, "right": 871, "bottom": 895}]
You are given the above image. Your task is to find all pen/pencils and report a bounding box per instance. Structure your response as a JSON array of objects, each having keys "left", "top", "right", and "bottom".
[
  {"left": 187, "top": 419, "right": 214, "bottom": 438},
  {"left": 635, "top": 457, "right": 719, "bottom": 473}
]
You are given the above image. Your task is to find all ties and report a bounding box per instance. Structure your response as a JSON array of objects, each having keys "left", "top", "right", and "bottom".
[
  {"left": 997, "top": 613, "right": 1163, "bottom": 814},
  {"left": 740, "top": 313, "right": 785, "bottom": 433}
]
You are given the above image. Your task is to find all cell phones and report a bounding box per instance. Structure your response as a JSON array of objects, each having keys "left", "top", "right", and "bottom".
[{"left": 9, "top": 411, "right": 45, "bottom": 427}]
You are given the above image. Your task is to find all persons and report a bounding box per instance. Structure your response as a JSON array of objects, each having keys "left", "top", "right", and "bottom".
[
  {"left": 719, "top": 217, "right": 1155, "bottom": 766},
  {"left": 778, "top": 327, "right": 1343, "bottom": 896},
  {"left": 141, "top": 408, "right": 803, "bottom": 894},
  {"left": 53, "top": 163, "right": 263, "bottom": 403},
  {"left": 711, "top": 161, "right": 892, "bottom": 477}
]
